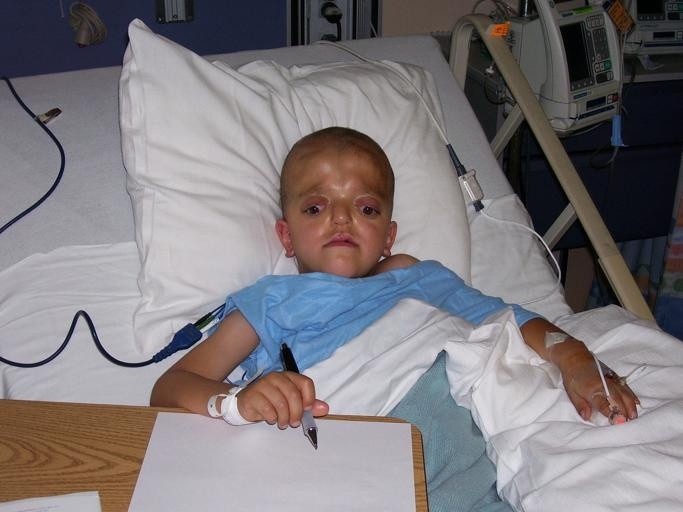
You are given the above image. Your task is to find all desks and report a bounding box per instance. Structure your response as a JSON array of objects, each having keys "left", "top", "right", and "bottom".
[{"left": 0, "top": 399, "right": 430, "bottom": 512}]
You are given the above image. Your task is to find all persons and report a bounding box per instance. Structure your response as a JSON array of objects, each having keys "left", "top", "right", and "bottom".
[{"left": 149, "top": 127, "right": 642, "bottom": 430}]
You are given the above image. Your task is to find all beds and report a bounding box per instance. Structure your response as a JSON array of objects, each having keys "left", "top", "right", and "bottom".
[{"left": 0, "top": 16, "right": 683, "bottom": 512}]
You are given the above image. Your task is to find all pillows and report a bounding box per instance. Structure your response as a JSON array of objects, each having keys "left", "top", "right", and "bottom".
[{"left": 119, "top": 17, "right": 471, "bottom": 354}]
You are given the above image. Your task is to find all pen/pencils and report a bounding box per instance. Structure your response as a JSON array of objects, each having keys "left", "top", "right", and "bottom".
[{"left": 281, "top": 343, "right": 319, "bottom": 451}]
